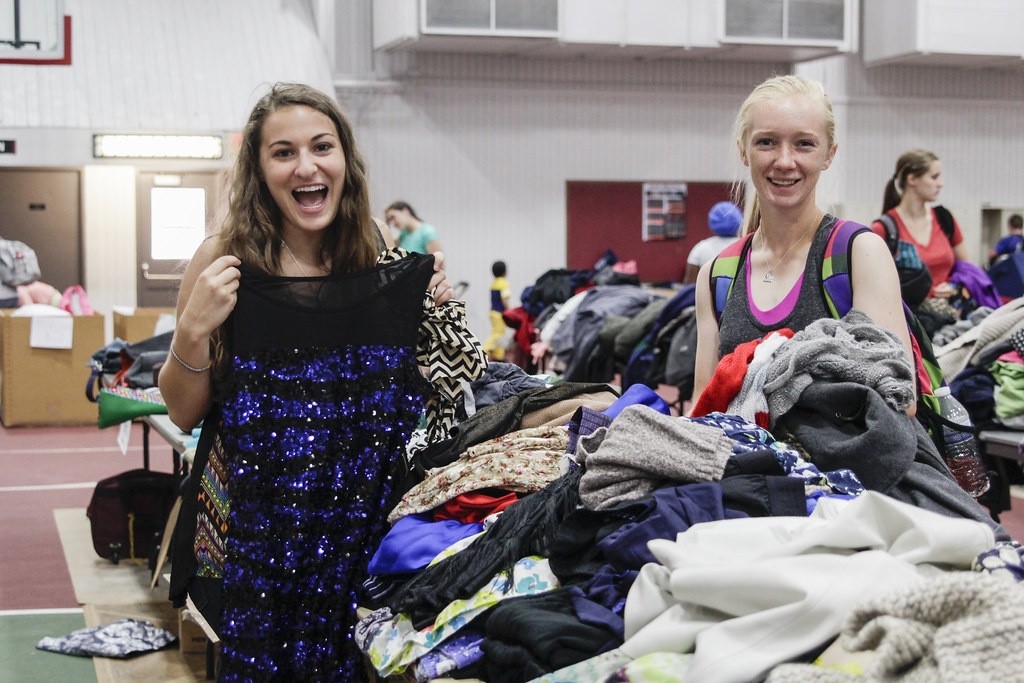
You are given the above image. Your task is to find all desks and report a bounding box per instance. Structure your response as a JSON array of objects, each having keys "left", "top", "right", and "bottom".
[{"left": 142, "top": 414, "right": 195, "bottom": 476}]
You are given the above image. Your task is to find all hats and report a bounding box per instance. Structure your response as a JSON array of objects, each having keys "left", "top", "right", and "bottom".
[{"left": 708, "top": 201, "right": 741, "bottom": 237}]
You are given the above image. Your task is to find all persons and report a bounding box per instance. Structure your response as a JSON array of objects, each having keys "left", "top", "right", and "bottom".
[
  {"left": 384, "top": 201, "right": 440, "bottom": 255},
  {"left": 870, "top": 149, "right": 970, "bottom": 298},
  {"left": 481, "top": 261, "right": 511, "bottom": 363},
  {"left": 690, "top": 74, "right": 918, "bottom": 420},
  {"left": 682, "top": 200, "right": 743, "bottom": 286},
  {"left": 17, "top": 280, "right": 62, "bottom": 308},
  {"left": 158, "top": 85, "right": 454, "bottom": 683},
  {"left": 990, "top": 214, "right": 1024, "bottom": 258}
]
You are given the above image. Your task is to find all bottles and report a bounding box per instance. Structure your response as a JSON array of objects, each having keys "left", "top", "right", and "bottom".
[{"left": 934, "top": 386, "right": 991, "bottom": 498}]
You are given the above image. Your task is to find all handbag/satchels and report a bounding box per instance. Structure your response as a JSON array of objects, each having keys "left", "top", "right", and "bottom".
[
  {"left": 86, "top": 469, "right": 185, "bottom": 564},
  {"left": 86, "top": 337, "right": 132, "bottom": 403}
]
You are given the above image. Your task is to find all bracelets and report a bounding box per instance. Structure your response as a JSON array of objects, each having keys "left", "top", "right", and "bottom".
[{"left": 171, "top": 347, "right": 211, "bottom": 373}]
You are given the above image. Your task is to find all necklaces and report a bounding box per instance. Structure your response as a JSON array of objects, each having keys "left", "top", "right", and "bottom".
[
  {"left": 760, "top": 220, "right": 813, "bottom": 282},
  {"left": 281, "top": 240, "right": 311, "bottom": 286}
]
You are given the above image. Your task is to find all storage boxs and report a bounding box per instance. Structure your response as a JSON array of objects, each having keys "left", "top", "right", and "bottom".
[
  {"left": 113, "top": 306, "right": 179, "bottom": 424},
  {"left": 0, "top": 307, "right": 106, "bottom": 429}
]
